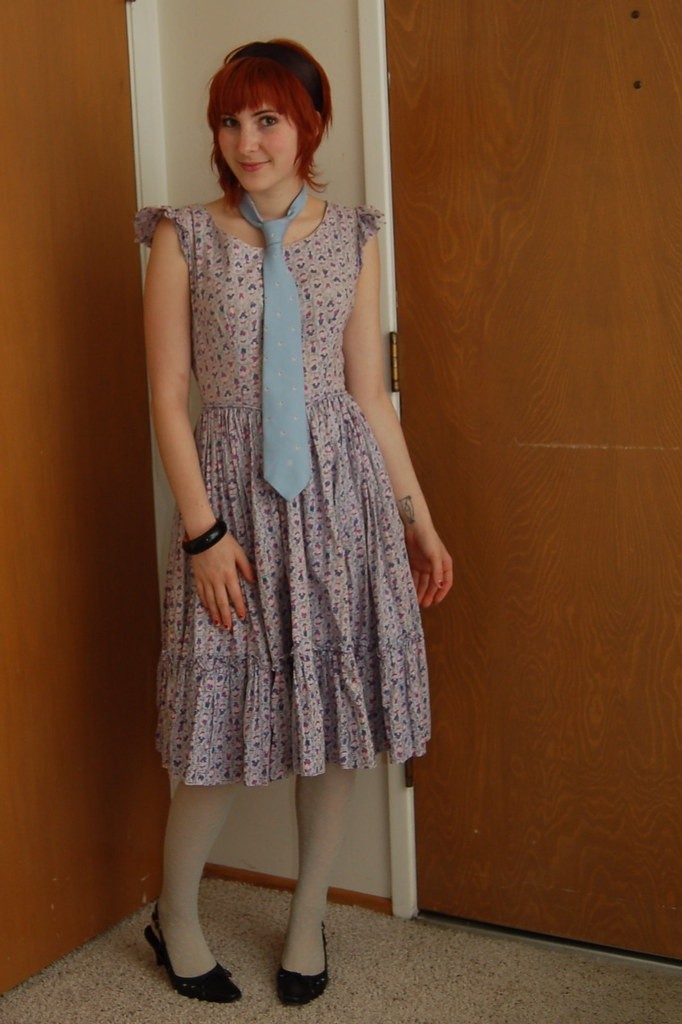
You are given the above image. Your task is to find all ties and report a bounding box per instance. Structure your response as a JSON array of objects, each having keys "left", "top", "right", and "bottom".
[{"left": 235, "top": 180, "right": 310, "bottom": 501}]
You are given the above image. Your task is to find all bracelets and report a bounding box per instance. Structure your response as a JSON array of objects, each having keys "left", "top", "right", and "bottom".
[{"left": 182, "top": 519, "right": 227, "bottom": 555}]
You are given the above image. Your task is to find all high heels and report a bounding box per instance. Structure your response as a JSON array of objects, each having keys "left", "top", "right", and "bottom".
[
  {"left": 144, "top": 901, "right": 242, "bottom": 1003},
  {"left": 277, "top": 921, "right": 328, "bottom": 1004}
]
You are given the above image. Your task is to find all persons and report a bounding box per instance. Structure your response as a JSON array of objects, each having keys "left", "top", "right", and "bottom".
[{"left": 135, "top": 38, "right": 453, "bottom": 1010}]
